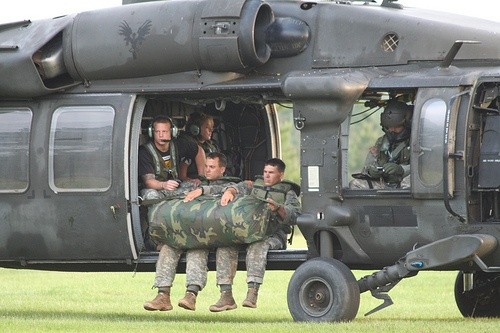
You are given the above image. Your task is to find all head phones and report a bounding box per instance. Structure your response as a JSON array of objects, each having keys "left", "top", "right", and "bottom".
[
  {"left": 190, "top": 113, "right": 207, "bottom": 136},
  {"left": 148, "top": 114, "right": 178, "bottom": 137}
]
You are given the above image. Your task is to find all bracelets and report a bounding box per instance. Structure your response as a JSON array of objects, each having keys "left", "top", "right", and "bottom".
[
  {"left": 161, "top": 181, "right": 164, "bottom": 190},
  {"left": 276, "top": 204, "right": 281, "bottom": 212}
]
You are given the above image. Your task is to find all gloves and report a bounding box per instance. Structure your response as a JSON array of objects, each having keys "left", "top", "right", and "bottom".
[
  {"left": 383, "top": 163, "right": 404, "bottom": 176},
  {"left": 368, "top": 167, "right": 383, "bottom": 178}
]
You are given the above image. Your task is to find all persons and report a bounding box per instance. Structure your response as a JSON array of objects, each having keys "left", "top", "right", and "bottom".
[
  {"left": 350, "top": 101, "right": 412, "bottom": 189},
  {"left": 144, "top": 152, "right": 237, "bottom": 311},
  {"left": 209, "top": 158, "right": 301, "bottom": 313},
  {"left": 139, "top": 116, "right": 201, "bottom": 250},
  {"left": 177, "top": 112, "right": 226, "bottom": 181}
]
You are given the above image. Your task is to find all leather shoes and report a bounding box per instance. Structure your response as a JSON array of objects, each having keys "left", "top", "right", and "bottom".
[
  {"left": 144, "top": 292, "right": 173, "bottom": 310},
  {"left": 154, "top": 242, "right": 165, "bottom": 252},
  {"left": 210, "top": 290, "right": 237, "bottom": 311},
  {"left": 243, "top": 288, "right": 258, "bottom": 308},
  {"left": 178, "top": 292, "right": 196, "bottom": 310}
]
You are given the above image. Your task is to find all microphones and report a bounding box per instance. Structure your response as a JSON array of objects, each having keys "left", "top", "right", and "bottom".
[{"left": 162, "top": 137, "right": 173, "bottom": 142}]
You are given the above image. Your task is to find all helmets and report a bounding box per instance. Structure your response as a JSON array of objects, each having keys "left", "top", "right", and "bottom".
[{"left": 381, "top": 101, "right": 413, "bottom": 142}]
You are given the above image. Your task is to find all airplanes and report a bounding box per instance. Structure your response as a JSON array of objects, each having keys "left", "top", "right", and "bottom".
[{"left": 0, "top": 0, "right": 500, "bottom": 323}]
